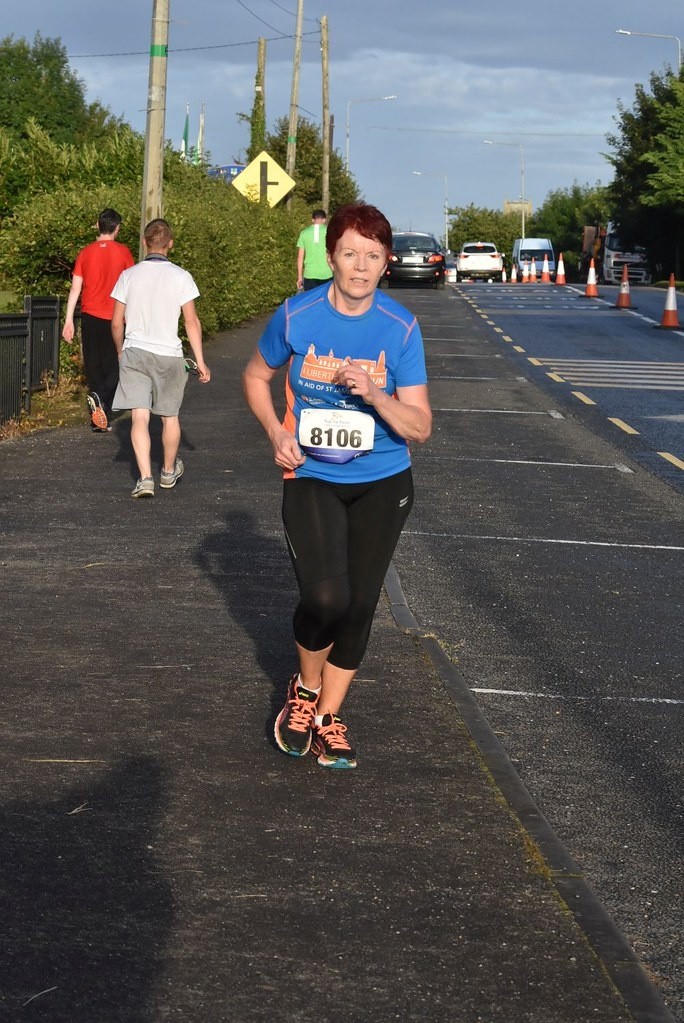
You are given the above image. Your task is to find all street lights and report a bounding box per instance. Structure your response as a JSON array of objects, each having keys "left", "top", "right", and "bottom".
[
  {"left": 614, "top": 29, "right": 682, "bottom": 81},
  {"left": 483, "top": 140, "right": 526, "bottom": 238},
  {"left": 413, "top": 171, "right": 449, "bottom": 250},
  {"left": 345, "top": 95, "right": 398, "bottom": 178}
]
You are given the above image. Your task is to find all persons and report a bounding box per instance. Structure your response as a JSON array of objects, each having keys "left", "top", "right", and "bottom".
[
  {"left": 110, "top": 219, "right": 211, "bottom": 496},
  {"left": 241, "top": 204, "right": 432, "bottom": 770},
  {"left": 62, "top": 208, "right": 134, "bottom": 432},
  {"left": 296, "top": 210, "right": 334, "bottom": 292}
]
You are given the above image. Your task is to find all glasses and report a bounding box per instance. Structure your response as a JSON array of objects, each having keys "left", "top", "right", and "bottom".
[{"left": 184, "top": 358, "right": 199, "bottom": 375}]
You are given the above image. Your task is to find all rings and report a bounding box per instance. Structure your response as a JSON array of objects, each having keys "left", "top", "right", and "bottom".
[{"left": 353, "top": 379, "right": 355, "bottom": 388}]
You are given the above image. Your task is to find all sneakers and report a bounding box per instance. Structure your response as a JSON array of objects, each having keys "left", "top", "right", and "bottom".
[
  {"left": 86, "top": 391, "right": 107, "bottom": 428},
  {"left": 92, "top": 421, "right": 111, "bottom": 432},
  {"left": 160, "top": 455, "right": 184, "bottom": 489},
  {"left": 273, "top": 672, "right": 321, "bottom": 757},
  {"left": 130, "top": 476, "right": 154, "bottom": 497},
  {"left": 310, "top": 707, "right": 356, "bottom": 769}
]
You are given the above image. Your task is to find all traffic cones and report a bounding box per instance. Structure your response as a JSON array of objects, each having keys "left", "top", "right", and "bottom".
[
  {"left": 510, "top": 264, "right": 517, "bottom": 283},
  {"left": 538, "top": 254, "right": 552, "bottom": 284},
  {"left": 609, "top": 264, "right": 638, "bottom": 309},
  {"left": 551, "top": 252, "right": 569, "bottom": 286},
  {"left": 579, "top": 258, "right": 604, "bottom": 298},
  {"left": 653, "top": 273, "right": 683, "bottom": 330},
  {"left": 502, "top": 268, "right": 507, "bottom": 283},
  {"left": 521, "top": 259, "right": 529, "bottom": 282},
  {"left": 526, "top": 257, "right": 540, "bottom": 283}
]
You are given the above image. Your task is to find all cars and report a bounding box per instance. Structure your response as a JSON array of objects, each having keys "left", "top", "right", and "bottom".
[
  {"left": 454, "top": 241, "right": 506, "bottom": 282},
  {"left": 379, "top": 235, "right": 444, "bottom": 290},
  {"left": 443, "top": 263, "right": 475, "bottom": 283}
]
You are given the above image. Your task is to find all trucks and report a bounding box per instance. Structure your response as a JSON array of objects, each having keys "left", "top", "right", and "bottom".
[{"left": 577, "top": 222, "right": 652, "bottom": 285}]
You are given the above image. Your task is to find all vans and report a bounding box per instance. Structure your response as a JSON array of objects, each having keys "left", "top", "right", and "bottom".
[{"left": 510, "top": 238, "right": 556, "bottom": 280}]
